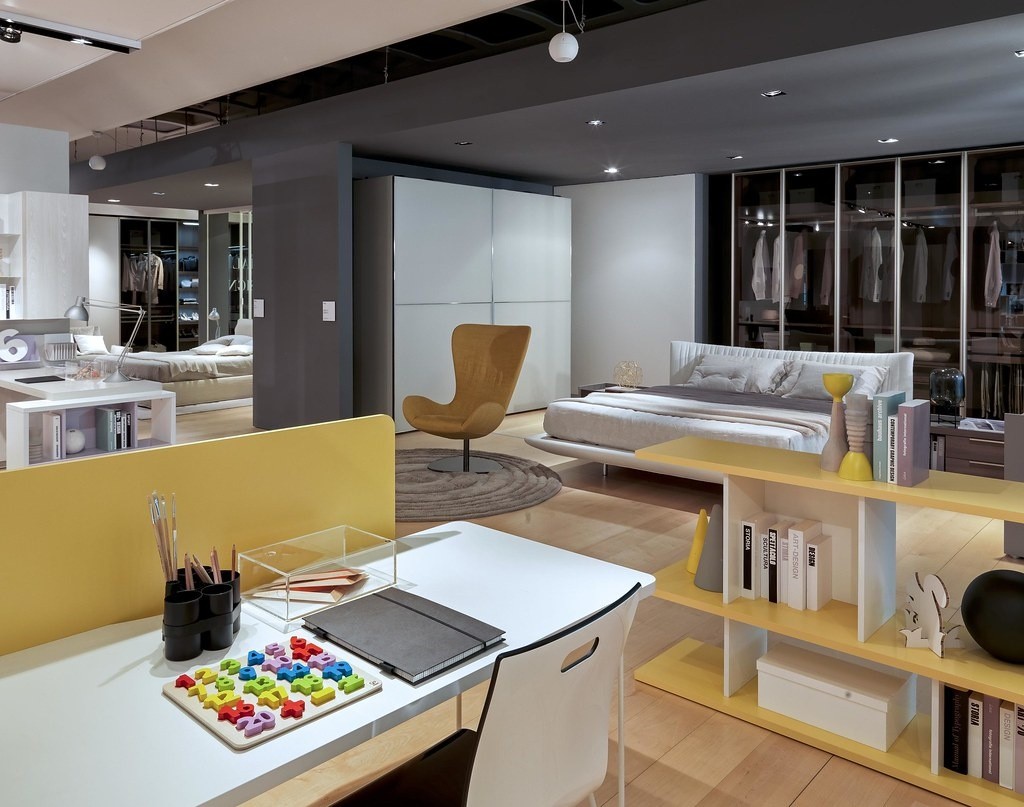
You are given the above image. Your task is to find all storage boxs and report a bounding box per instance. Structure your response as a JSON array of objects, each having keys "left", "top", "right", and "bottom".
[{"left": 756, "top": 639, "right": 917, "bottom": 751}]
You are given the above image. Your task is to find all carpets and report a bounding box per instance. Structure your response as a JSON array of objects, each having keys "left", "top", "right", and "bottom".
[{"left": 396, "top": 449, "right": 562, "bottom": 523}]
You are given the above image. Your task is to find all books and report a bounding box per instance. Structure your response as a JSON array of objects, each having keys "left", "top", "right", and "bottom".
[{"left": 302, "top": 587, "right": 506, "bottom": 686}]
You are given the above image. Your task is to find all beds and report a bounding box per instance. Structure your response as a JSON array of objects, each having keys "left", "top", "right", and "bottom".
[
  {"left": 524, "top": 340, "right": 913, "bottom": 478},
  {"left": 73, "top": 318, "right": 253, "bottom": 420}
]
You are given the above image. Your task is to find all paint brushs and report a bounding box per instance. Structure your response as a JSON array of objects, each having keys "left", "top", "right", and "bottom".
[
  {"left": 161, "top": 495, "right": 173, "bottom": 581},
  {"left": 152, "top": 490, "right": 170, "bottom": 581},
  {"left": 172, "top": 493, "right": 178, "bottom": 580},
  {"left": 146, "top": 495, "right": 168, "bottom": 582}
]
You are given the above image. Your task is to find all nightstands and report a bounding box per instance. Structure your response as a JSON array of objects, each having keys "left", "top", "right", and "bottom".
[
  {"left": 578, "top": 382, "right": 647, "bottom": 397},
  {"left": 930, "top": 413, "right": 1004, "bottom": 479}
]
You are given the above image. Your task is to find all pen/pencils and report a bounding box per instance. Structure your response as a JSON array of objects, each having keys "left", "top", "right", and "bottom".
[
  {"left": 192, "top": 554, "right": 213, "bottom": 584},
  {"left": 213, "top": 546, "right": 222, "bottom": 584},
  {"left": 210, "top": 551, "right": 218, "bottom": 584},
  {"left": 191, "top": 562, "right": 208, "bottom": 584},
  {"left": 186, "top": 558, "right": 195, "bottom": 590},
  {"left": 184, "top": 554, "right": 190, "bottom": 590},
  {"left": 232, "top": 544, "right": 236, "bottom": 581}
]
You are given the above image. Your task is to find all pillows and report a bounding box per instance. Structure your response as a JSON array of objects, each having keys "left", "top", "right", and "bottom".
[
  {"left": 683, "top": 354, "right": 891, "bottom": 400},
  {"left": 0, "top": 325, "right": 109, "bottom": 371},
  {"left": 189, "top": 334, "right": 254, "bottom": 356}
]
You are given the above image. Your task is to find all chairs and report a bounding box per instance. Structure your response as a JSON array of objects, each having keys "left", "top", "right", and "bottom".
[
  {"left": 325, "top": 581, "right": 642, "bottom": 807},
  {"left": 402, "top": 326, "right": 530, "bottom": 473}
]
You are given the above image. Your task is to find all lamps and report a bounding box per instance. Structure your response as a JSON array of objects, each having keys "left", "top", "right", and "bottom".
[
  {"left": 209, "top": 307, "right": 221, "bottom": 339},
  {"left": 0, "top": 17, "right": 21, "bottom": 43},
  {"left": 89, "top": 130, "right": 106, "bottom": 171},
  {"left": 548, "top": 0, "right": 585, "bottom": 62},
  {"left": 64, "top": 297, "right": 147, "bottom": 384}
]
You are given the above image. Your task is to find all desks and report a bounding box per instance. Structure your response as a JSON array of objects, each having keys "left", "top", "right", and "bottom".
[
  {"left": 0, "top": 369, "right": 162, "bottom": 457},
  {"left": 1, "top": 413, "right": 656, "bottom": 807}
]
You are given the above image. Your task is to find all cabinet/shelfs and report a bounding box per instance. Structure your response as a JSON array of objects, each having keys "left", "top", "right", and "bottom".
[
  {"left": 6, "top": 390, "right": 176, "bottom": 472},
  {"left": 197, "top": 207, "right": 253, "bottom": 345},
  {"left": 0, "top": 191, "right": 203, "bottom": 351},
  {"left": 728, "top": 145, "right": 1024, "bottom": 479},
  {"left": 633, "top": 436, "right": 1024, "bottom": 807},
  {"left": 352, "top": 173, "right": 573, "bottom": 434}
]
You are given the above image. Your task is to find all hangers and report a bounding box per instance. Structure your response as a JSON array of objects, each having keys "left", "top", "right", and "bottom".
[{"left": 760, "top": 209, "right": 1020, "bottom": 233}]
